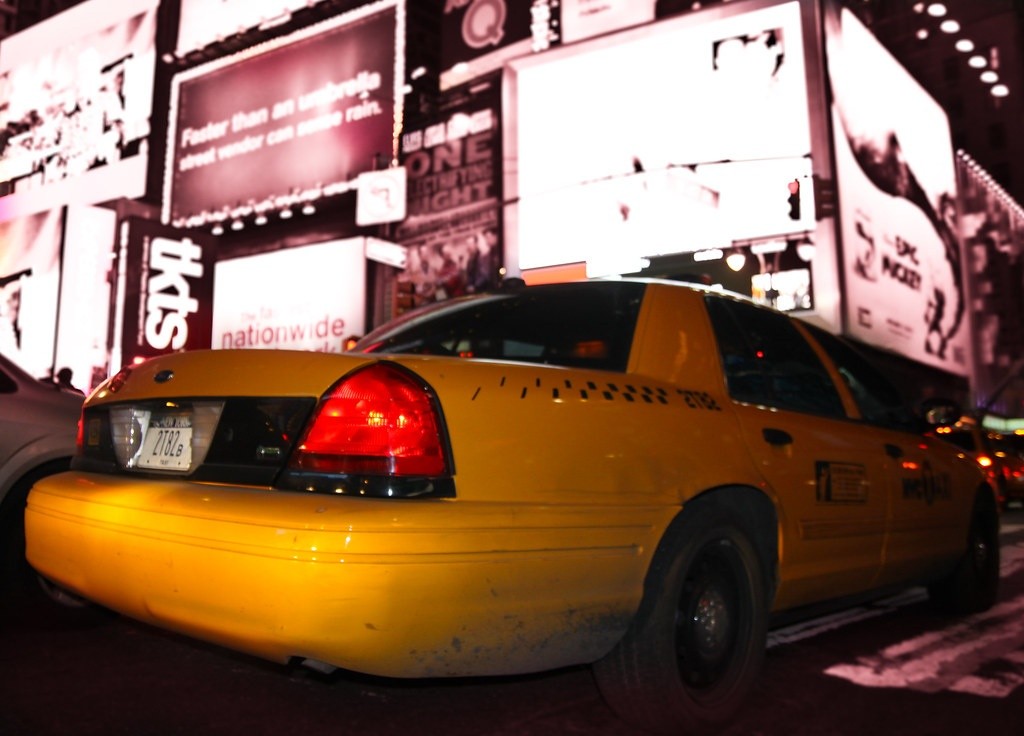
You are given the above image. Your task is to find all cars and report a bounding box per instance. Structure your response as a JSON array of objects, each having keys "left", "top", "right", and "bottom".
[
  {"left": 0, "top": 351, "right": 86, "bottom": 554},
  {"left": 946, "top": 427, "right": 1023, "bottom": 508},
  {"left": 24, "top": 274, "right": 998, "bottom": 736}
]
